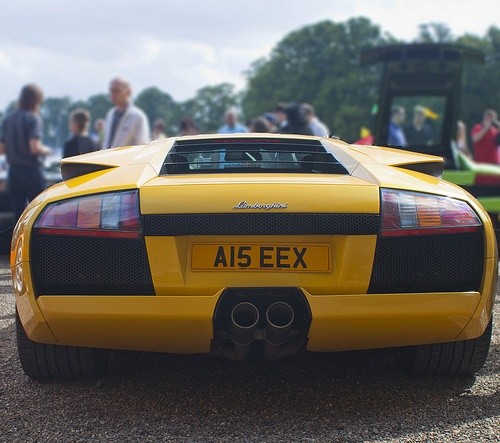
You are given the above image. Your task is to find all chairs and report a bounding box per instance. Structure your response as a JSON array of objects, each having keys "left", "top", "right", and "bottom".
[
  {"left": 301, "top": 156, "right": 327, "bottom": 172},
  {"left": 168, "top": 156, "right": 189, "bottom": 172}
]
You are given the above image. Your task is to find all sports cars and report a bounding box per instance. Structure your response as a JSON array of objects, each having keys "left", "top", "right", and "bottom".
[{"left": 9, "top": 133, "right": 497, "bottom": 382}]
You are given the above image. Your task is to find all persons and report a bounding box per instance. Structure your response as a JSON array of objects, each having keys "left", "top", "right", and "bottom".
[
  {"left": 0, "top": 84, "right": 54, "bottom": 230},
  {"left": 94, "top": 75, "right": 330, "bottom": 151},
  {"left": 61, "top": 107, "right": 100, "bottom": 181},
  {"left": 387, "top": 103, "right": 500, "bottom": 166}
]
